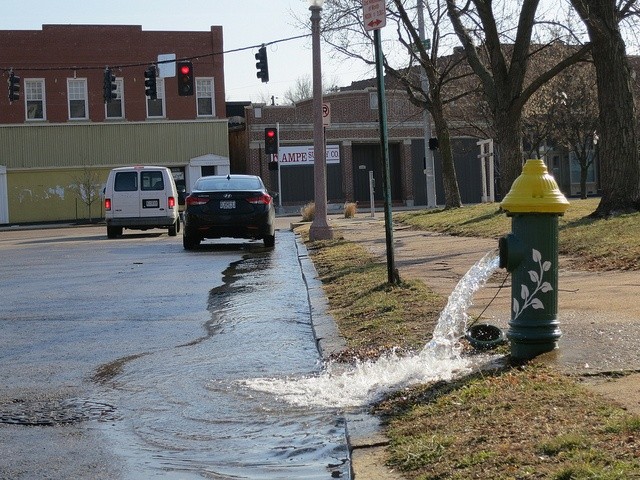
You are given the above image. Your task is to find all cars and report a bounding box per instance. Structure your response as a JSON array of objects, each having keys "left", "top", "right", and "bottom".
[{"left": 183, "top": 176, "right": 275, "bottom": 250}]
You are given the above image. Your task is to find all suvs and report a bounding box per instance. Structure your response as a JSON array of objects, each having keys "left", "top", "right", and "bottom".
[{"left": 104, "top": 166, "right": 180, "bottom": 238}]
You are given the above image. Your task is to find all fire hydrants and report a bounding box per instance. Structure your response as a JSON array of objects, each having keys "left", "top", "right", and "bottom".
[{"left": 497, "top": 161, "right": 569, "bottom": 359}]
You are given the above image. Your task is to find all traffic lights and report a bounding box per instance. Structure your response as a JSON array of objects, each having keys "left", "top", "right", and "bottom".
[
  {"left": 103, "top": 70, "right": 117, "bottom": 103},
  {"left": 178, "top": 61, "right": 193, "bottom": 95},
  {"left": 7, "top": 71, "right": 19, "bottom": 102},
  {"left": 265, "top": 128, "right": 277, "bottom": 154},
  {"left": 144, "top": 65, "right": 157, "bottom": 100},
  {"left": 256, "top": 46, "right": 269, "bottom": 83}
]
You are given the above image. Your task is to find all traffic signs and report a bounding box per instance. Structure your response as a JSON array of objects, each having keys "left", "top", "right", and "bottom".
[
  {"left": 323, "top": 103, "right": 331, "bottom": 126},
  {"left": 362, "top": 0, "right": 386, "bottom": 31}
]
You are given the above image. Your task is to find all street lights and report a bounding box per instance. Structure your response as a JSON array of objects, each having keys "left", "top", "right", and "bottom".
[{"left": 308, "top": 0, "right": 333, "bottom": 243}]
